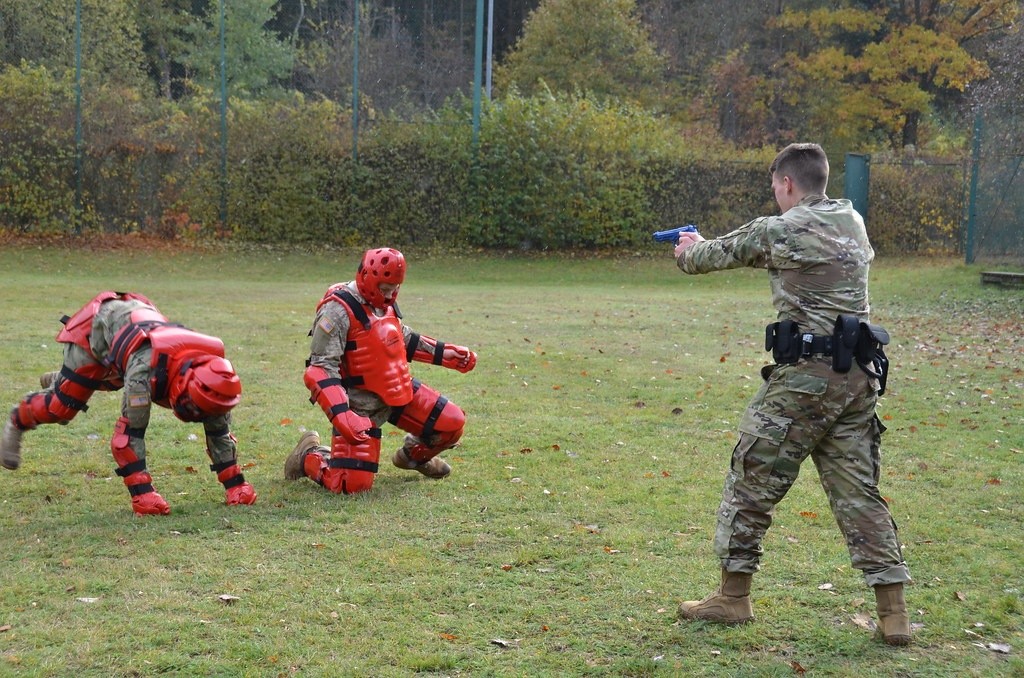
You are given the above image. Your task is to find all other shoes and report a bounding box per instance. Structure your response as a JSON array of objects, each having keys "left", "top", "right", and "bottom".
[
  {"left": 284, "top": 431, "right": 320, "bottom": 480},
  {"left": 0, "top": 423, "right": 21, "bottom": 470},
  {"left": 392, "top": 447, "right": 451, "bottom": 478}
]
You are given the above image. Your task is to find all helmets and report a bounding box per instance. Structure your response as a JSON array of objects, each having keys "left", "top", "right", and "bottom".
[
  {"left": 170, "top": 355, "right": 241, "bottom": 422},
  {"left": 356, "top": 248, "right": 406, "bottom": 310}
]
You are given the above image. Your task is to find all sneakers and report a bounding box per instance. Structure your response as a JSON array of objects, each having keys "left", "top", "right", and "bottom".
[{"left": 40, "top": 372, "right": 69, "bottom": 425}]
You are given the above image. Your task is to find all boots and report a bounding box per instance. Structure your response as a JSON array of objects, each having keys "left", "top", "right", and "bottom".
[
  {"left": 873, "top": 583, "right": 913, "bottom": 646},
  {"left": 678, "top": 569, "right": 754, "bottom": 625}
]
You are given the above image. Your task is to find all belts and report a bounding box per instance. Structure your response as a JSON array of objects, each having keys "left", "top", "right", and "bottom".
[{"left": 799, "top": 334, "right": 835, "bottom": 355}]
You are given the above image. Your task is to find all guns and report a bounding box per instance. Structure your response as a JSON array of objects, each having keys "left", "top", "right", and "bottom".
[{"left": 652, "top": 225, "right": 697, "bottom": 247}]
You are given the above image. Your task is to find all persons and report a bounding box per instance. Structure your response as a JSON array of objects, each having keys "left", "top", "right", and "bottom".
[
  {"left": 675, "top": 140, "right": 914, "bottom": 644},
  {"left": 0, "top": 290, "right": 257, "bottom": 515},
  {"left": 282, "top": 246, "right": 477, "bottom": 496}
]
